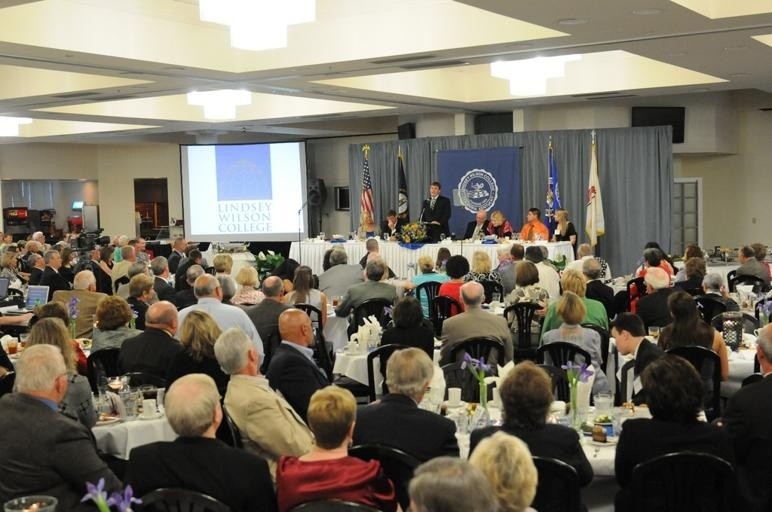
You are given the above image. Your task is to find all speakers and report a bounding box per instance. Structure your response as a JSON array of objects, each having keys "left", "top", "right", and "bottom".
[{"left": 307, "top": 178, "right": 325, "bottom": 206}]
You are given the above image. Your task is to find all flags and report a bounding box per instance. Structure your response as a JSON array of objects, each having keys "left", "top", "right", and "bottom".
[
  {"left": 584, "top": 142, "right": 607, "bottom": 247},
  {"left": 544, "top": 136, "right": 566, "bottom": 233},
  {"left": 396, "top": 153, "right": 412, "bottom": 231},
  {"left": 359, "top": 156, "right": 376, "bottom": 227}
]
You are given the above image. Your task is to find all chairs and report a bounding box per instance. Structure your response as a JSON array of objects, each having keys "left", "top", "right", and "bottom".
[{"left": 1, "top": 259, "right": 771, "bottom": 512}]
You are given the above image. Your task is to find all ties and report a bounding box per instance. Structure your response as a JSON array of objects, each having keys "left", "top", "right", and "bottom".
[{"left": 528, "top": 224, "right": 534, "bottom": 240}]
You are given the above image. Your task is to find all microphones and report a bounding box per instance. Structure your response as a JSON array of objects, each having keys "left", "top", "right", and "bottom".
[{"left": 423, "top": 198, "right": 431, "bottom": 208}]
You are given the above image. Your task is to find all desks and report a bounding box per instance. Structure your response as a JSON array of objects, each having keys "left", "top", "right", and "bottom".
[{"left": 287, "top": 234, "right": 577, "bottom": 284}]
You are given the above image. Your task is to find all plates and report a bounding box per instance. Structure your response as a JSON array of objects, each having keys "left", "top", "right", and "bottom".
[
  {"left": 344, "top": 351, "right": 360, "bottom": 356},
  {"left": 550, "top": 393, "right": 640, "bottom": 448}
]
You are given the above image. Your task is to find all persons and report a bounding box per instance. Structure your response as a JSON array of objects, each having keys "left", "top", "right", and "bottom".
[
  {"left": 418, "top": 180, "right": 452, "bottom": 244},
  {"left": 0, "top": 209, "right": 772, "bottom": 511}
]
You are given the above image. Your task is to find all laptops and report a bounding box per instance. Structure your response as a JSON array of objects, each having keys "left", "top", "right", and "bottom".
[
  {"left": 7, "top": 285, "right": 50, "bottom": 314},
  {"left": 0, "top": 276, "right": 10, "bottom": 300}
]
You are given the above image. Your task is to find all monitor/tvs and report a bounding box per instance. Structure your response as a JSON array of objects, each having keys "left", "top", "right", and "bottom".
[{"left": 72, "top": 200, "right": 85, "bottom": 211}]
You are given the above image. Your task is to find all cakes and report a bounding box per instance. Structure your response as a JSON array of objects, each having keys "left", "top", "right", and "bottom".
[{"left": 592, "top": 425, "right": 607, "bottom": 442}]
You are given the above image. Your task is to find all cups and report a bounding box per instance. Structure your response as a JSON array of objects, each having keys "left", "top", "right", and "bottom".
[
  {"left": 479, "top": 229, "right": 484, "bottom": 240},
  {"left": 319, "top": 232, "right": 326, "bottom": 239},
  {"left": 8, "top": 337, "right": 18, "bottom": 354},
  {"left": 347, "top": 340, "right": 357, "bottom": 352},
  {"left": 440, "top": 234, "right": 445, "bottom": 242},
  {"left": 384, "top": 233, "right": 389, "bottom": 240},
  {"left": 491, "top": 292, "right": 501, "bottom": 306},
  {"left": 332, "top": 235, "right": 343, "bottom": 240},
  {"left": 660, "top": 326, "right": 666, "bottom": 333},
  {"left": 722, "top": 311, "right": 742, "bottom": 344},
  {"left": 20, "top": 333, "right": 31, "bottom": 347},
  {"left": 736, "top": 283, "right": 754, "bottom": 307},
  {"left": 331, "top": 296, "right": 339, "bottom": 307},
  {"left": 510, "top": 232, "right": 544, "bottom": 240},
  {"left": 648, "top": 326, "right": 659, "bottom": 338},
  {"left": 83, "top": 371, "right": 167, "bottom": 426},
  {"left": 439, "top": 386, "right": 487, "bottom": 440}
]
